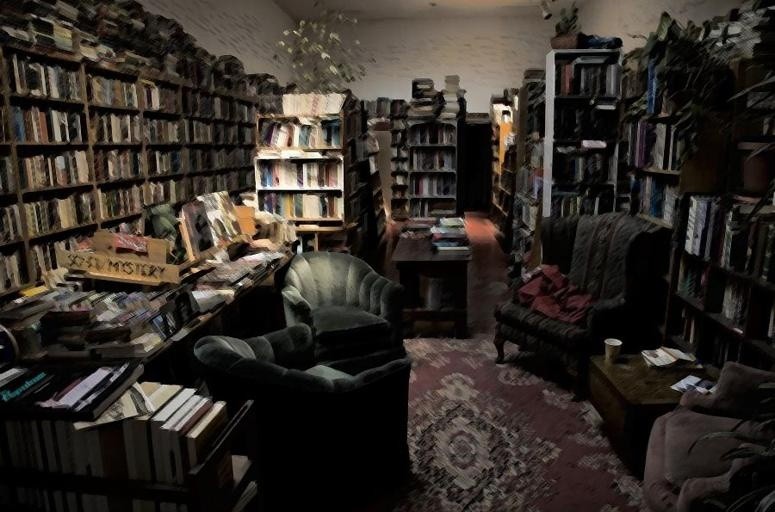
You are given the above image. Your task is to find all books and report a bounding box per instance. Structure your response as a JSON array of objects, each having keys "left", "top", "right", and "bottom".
[
  {"left": 344, "top": 78, "right": 470, "bottom": 251},
  {"left": 258, "top": 119, "right": 342, "bottom": 219},
  {"left": 551, "top": 56, "right": 621, "bottom": 218},
  {"left": 0, "top": 0, "right": 257, "bottom": 290},
  {"left": 490, "top": 68, "right": 545, "bottom": 257},
  {"left": 620, "top": 61, "right": 775, "bottom": 376},
  {"left": 0, "top": 191, "right": 284, "bottom": 512}
]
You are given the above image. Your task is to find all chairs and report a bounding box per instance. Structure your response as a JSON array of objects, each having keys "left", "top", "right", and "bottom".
[
  {"left": 643, "top": 364, "right": 775, "bottom": 512},
  {"left": 494, "top": 213, "right": 668, "bottom": 402}
]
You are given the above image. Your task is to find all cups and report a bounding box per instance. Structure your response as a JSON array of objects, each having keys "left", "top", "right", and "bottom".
[{"left": 604, "top": 338, "right": 623, "bottom": 364}]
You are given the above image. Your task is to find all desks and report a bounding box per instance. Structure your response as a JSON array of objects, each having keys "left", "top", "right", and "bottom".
[
  {"left": 3, "top": 221, "right": 301, "bottom": 511},
  {"left": 589, "top": 356, "right": 717, "bottom": 475},
  {"left": 391, "top": 225, "right": 472, "bottom": 338}
]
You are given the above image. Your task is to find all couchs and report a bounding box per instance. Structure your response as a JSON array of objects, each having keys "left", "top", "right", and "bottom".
[
  {"left": 193, "top": 320, "right": 414, "bottom": 512},
  {"left": 273, "top": 248, "right": 403, "bottom": 357}
]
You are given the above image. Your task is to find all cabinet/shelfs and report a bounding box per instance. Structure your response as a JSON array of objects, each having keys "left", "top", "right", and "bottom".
[
  {"left": 391, "top": 90, "right": 456, "bottom": 213},
  {"left": 491, "top": 47, "right": 774, "bottom": 362},
  {"left": 256, "top": 85, "right": 390, "bottom": 262},
  {"left": 0, "top": 29, "right": 264, "bottom": 288}
]
